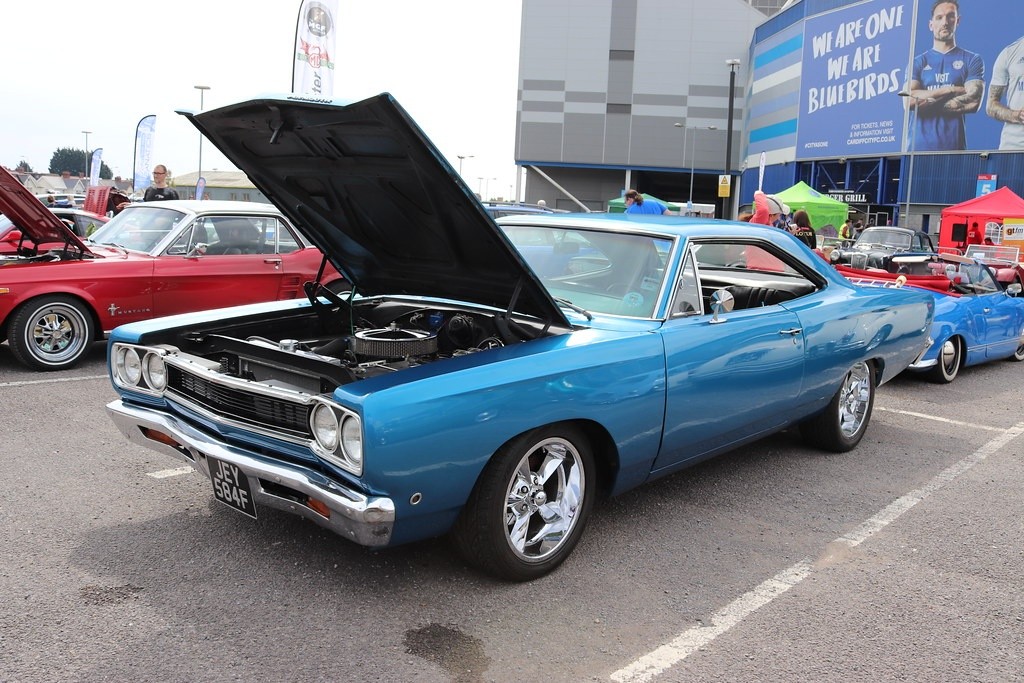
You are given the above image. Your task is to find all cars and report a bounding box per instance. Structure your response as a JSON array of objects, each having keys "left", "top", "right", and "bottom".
[
  {"left": 103, "top": 91, "right": 937, "bottom": 584},
  {"left": 0, "top": 165, "right": 362, "bottom": 373},
  {"left": 833, "top": 253, "right": 1024, "bottom": 384},
  {"left": 831, "top": 227, "right": 939, "bottom": 275},
  {"left": 0, "top": 194, "right": 86, "bottom": 221},
  {"left": 483, "top": 204, "right": 671, "bottom": 277},
  {"left": 0, "top": 208, "right": 114, "bottom": 256}
]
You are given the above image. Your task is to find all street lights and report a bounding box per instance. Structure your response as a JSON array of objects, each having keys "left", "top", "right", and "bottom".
[
  {"left": 81, "top": 131, "right": 92, "bottom": 186},
  {"left": 195, "top": 85, "right": 210, "bottom": 178},
  {"left": 478, "top": 177, "right": 497, "bottom": 202}
]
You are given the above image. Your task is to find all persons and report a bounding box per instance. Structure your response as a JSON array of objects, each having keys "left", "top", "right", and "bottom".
[
  {"left": 986, "top": 35, "right": 1024, "bottom": 150},
  {"left": 903, "top": 0, "right": 984, "bottom": 151},
  {"left": 47, "top": 195, "right": 76, "bottom": 207},
  {"left": 106, "top": 197, "right": 116, "bottom": 218},
  {"left": 839, "top": 217, "right": 865, "bottom": 251},
  {"left": 624, "top": 190, "right": 672, "bottom": 252},
  {"left": 190, "top": 193, "right": 210, "bottom": 200},
  {"left": 141, "top": 165, "right": 179, "bottom": 242},
  {"left": 738, "top": 190, "right": 816, "bottom": 273},
  {"left": 967, "top": 221, "right": 996, "bottom": 258}
]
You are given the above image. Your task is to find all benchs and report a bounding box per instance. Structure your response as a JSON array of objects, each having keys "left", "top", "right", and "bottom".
[
  {"left": 837, "top": 264, "right": 963, "bottom": 297},
  {"left": 207, "top": 241, "right": 286, "bottom": 256}
]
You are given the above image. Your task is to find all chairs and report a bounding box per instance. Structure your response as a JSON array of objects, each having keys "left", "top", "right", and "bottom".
[{"left": 718, "top": 284, "right": 798, "bottom": 311}]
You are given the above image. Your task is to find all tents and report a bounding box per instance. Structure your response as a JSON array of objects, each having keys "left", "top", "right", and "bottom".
[
  {"left": 607, "top": 192, "right": 681, "bottom": 216},
  {"left": 752, "top": 181, "right": 848, "bottom": 249},
  {"left": 938, "top": 185, "right": 1024, "bottom": 262},
  {"left": 668, "top": 202, "right": 716, "bottom": 219}
]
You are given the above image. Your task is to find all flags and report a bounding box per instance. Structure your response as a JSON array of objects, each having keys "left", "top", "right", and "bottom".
[
  {"left": 90, "top": 148, "right": 103, "bottom": 187},
  {"left": 291, "top": 0, "right": 338, "bottom": 104},
  {"left": 195, "top": 177, "right": 206, "bottom": 200},
  {"left": 133, "top": 115, "right": 156, "bottom": 192}
]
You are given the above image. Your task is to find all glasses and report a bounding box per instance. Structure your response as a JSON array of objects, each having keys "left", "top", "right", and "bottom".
[{"left": 152, "top": 172, "right": 165, "bottom": 175}]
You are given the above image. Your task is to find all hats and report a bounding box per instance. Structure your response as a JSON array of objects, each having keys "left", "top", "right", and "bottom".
[
  {"left": 845, "top": 219, "right": 852, "bottom": 223},
  {"left": 766, "top": 194, "right": 790, "bottom": 215}
]
